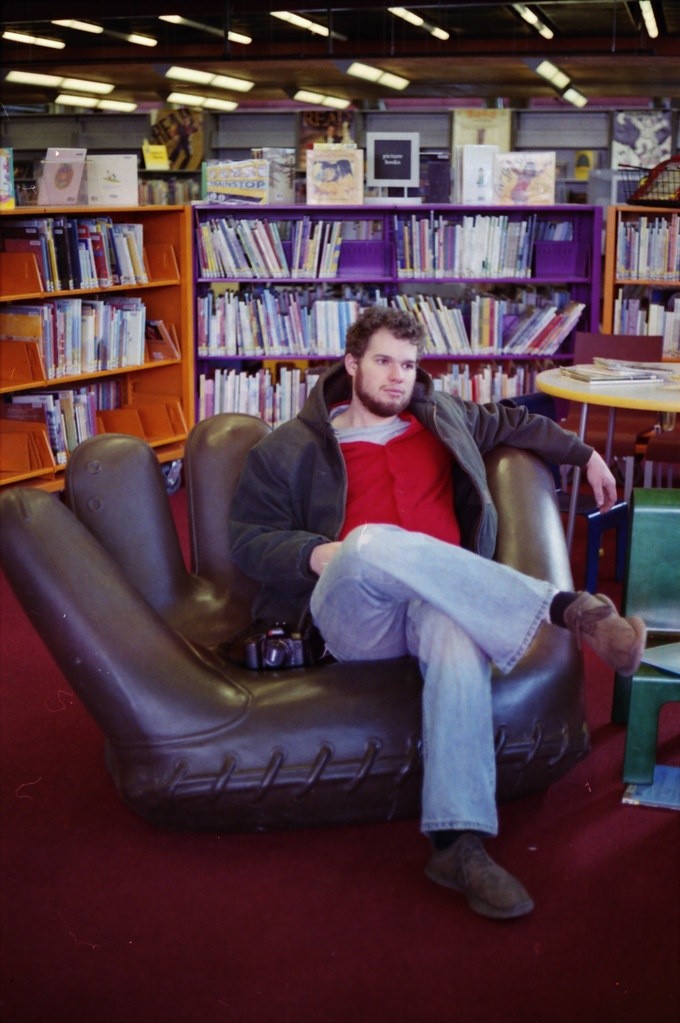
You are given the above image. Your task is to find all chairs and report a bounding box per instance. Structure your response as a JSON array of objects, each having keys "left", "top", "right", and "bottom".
[{"left": 497, "top": 328, "right": 680, "bottom": 814}]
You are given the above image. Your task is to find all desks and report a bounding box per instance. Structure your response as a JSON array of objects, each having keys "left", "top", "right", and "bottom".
[{"left": 533, "top": 358, "right": 680, "bottom": 595}]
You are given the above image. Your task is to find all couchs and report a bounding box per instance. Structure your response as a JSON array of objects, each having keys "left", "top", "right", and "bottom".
[{"left": 0, "top": 410, "right": 582, "bottom": 828}]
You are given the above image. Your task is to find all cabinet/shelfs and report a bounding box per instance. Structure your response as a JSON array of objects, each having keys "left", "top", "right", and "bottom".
[
  {"left": 0, "top": 198, "right": 193, "bottom": 491},
  {"left": 602, "top": 204, "right": 680, "bottom": 447},
  {"left": 194, "top": 197, "right": 604, "bottom": 423}
]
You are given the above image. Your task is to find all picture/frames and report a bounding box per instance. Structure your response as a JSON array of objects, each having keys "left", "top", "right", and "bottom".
[{"left": 365, "top": 128, "right": 420, "bottom": 187}]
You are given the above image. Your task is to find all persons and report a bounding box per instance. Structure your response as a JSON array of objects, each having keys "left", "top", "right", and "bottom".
[
  {"left": 305, "top": 143, "right": 364, "bottom": 204},
  {"left": 227, "top": 307, "right": 648, "bottom": 917},
  {"left": 169, "top": 118, "right": 198, "bottom": 169},
  {"left": 323, "top": 125, "right": 340, "bottom": 143}
]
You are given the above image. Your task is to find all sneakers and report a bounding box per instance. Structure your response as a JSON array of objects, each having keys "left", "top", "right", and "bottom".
[
  {"left": 565, "top": 591, "right": 646, "bottom": 677},
  {"left": 421, "top": 827, "right": 534, "bottom": 919}
]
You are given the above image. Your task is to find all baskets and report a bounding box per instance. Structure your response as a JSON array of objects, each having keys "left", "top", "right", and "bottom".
[{"left": 617, "top": 162, "right": 680, "bottom": 209}]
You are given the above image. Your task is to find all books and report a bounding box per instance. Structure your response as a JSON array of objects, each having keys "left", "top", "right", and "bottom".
[
  {"left": 38, "top": 148, "right": 138, "bottom": 206},
  {"left": 622, "top": 765, "right": 680, "bottom": 810},
  {"left": 0, "top": 148, "right": 16, "bottom": 209},
  {"left": 615, "top": 210, "right": 680, "bottom": 282},
  {"left": 206, "top": 148, "right": 295, "bottom": 204},
  {"left": 0, "top": 298, "right": 146, "bottom": 379},
  {"left": 540, "top": 219, "right": 573, "bottom": 240},
  {"left": 393, "top": 211, "right": 536, "bottom": 279},
  {"left": 12, "top": 380, "right": 117, "bottom": 465},
  {"left": 455, "top": 144, "right": 555, "bottom": 204},
  {"left": 200, "top": 216, "right": 373, "bottom": 278},
  {"left": 197, "top": 285, "right": 586, "bottom": 355},
  {"left": 15, "top": 160, "right": 40, "bottom": 206},
  {"left": 561, "top": 357, "right": 676, "bottom": 383},
  {"left": 138, "top": 180, "right": 199, "bottom": 204},
  {"left": 199, "top": 367, "right": 320, "bottom": 431},
  {"left": 0, "top": 217, "right": 149, "bottom": 292},
  {"left": 428, "top": 360, "right": 555, "bottom": 405},
  {"left": 613, "top": 288, "right": 680, "bottom": 362}
]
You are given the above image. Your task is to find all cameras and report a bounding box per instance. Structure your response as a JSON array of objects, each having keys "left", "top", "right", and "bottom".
[{"left": 242, "top": 627, "right": 308, "bottom": 673}]
